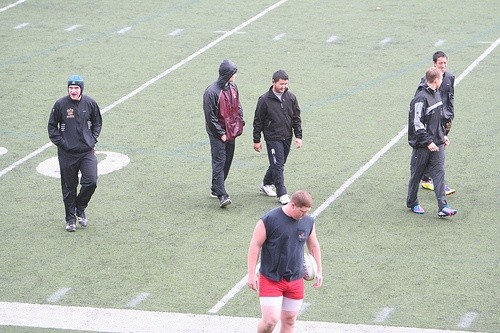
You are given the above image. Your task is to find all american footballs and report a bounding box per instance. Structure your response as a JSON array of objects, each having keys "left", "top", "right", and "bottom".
[{"left": 303, "top": 252, "right": 318, "bottom": 281}]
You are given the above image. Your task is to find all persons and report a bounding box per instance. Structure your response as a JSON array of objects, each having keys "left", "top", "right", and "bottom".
[
  {"left": 406, "top": 67, "right": 457, "bottom": 218},
  {"left": 253, "top": 70, "right": 303, "bottom": 205},
  {"left": 414, "top": 50, "right": 456, "bottom": 195},
  {"left": 203, "top": 59, "right": 245, "bottom": 207},
  {"left": 48, "top": 75, "right": 103, "bottom": 231},
  {"left": 246, "top": 190, "right": 323, "bottom": 333}
]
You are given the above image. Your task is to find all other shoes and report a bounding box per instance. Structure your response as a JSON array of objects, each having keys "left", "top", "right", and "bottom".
[
  {"left": 259, "top": 181, "right": 277, "bottom": 197},
  {"left": 210, "top": 191, "right": 231, "bottom": 199},
  {"left": 421, "top": 179, "right": 434, "bottom": 191},
  {"left": 438, "top": 206, "right": 457, "bottom": 217},
  {"left": 445, "top": 184, "right": 456, "bottom": 195},
  {"left": 218, "top": 194, "right": 231, "bottom": 208},
  {"left": 411, "top": 205, "right": 425, "bottom": 214},
  {"left": 277, "top": 194, "right": 291, "bottom": 205},
  {"left": 65, "top": 220, "right": 76, "bottom": 232},
  {"left": 76, "top": 209, "right": 88, "bottom": 228}
]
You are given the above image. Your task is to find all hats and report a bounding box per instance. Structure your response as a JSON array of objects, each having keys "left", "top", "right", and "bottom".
[{"left": 67, "top": 74, "right": 85, "bottom": 97}]
